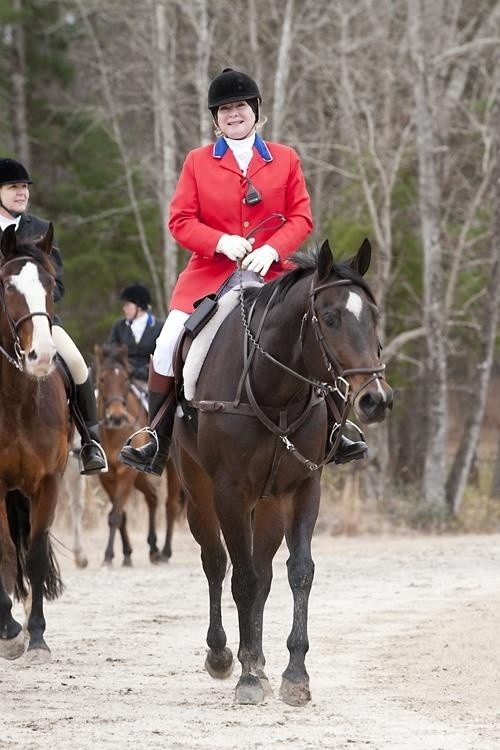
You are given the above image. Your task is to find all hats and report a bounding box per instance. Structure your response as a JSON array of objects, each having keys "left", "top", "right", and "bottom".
[{"left": 208, "top": 68, "right": 261, "bottom": 109}]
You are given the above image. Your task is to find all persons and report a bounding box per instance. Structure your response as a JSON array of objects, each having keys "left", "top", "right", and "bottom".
[
  {"left": 120, "top": 69, "right": 369, "bottom": 476},
  {"left": 0, "top": 156, "right": 106, "bottom": 472},
  {"left": 108, "top": 285, "right": 164, "bottom": 382}
]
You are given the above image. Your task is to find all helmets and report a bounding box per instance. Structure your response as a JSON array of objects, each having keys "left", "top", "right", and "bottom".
[
  {"left": 0, "top": 157, "right": 33, "bottom": 186},
  {"left": 119, "top": 282, "right": 151, "bottom": 311}
]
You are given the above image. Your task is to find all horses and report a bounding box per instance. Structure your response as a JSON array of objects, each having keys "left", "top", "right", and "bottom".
[
  {"left": 171, "top": 237, "right": 394, "bottom": 708},
  {"left": 94, "top": 342, "right": 186, "bottom": 569},
  {"left": 0, "top": 221, "right": 76, "bottom": 666}
]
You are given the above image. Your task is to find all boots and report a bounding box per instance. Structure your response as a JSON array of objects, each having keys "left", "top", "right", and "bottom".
[
  {"left": 120, "top": 353, "right": 178, "bottom": 474},
  {"left": 324, "top": 391, "right": 369, "bottom": 461},
  {"left": 70, "top": 364, "right": 106, "bottom": 471}
]
